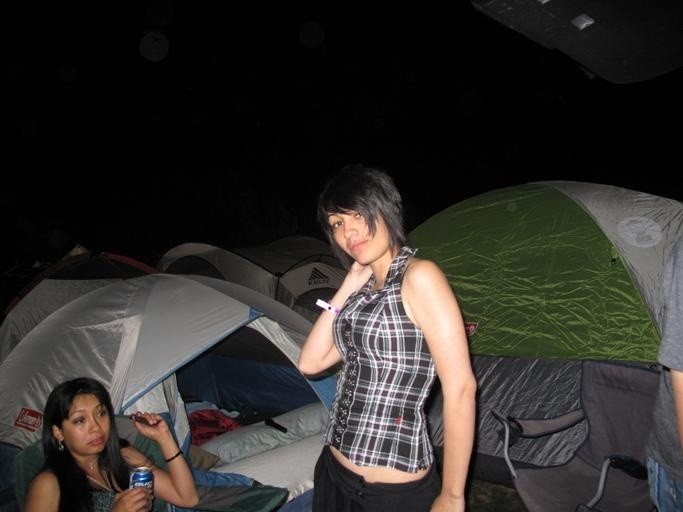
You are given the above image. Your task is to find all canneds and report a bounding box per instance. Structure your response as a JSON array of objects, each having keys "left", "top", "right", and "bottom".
[{"left": 130, "top": 466, "right": 153, "bottom": 497}]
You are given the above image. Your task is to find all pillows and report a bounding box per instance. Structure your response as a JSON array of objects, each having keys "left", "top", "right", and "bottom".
[{"left": 204, "top": 403, "right": 330, "bottom": 467}]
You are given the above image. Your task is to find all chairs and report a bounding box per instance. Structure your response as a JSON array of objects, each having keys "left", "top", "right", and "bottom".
[
  {"left": 10, "top": 417, "right": 288, "bottom": 511},
  {"left": 487, "top": 355, "right": 668, "bottom": 512}
]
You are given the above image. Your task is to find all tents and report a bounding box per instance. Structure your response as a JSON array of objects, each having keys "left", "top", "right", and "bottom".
[{"left": 405, "top": 181, "right": 682, "bottom": 511}]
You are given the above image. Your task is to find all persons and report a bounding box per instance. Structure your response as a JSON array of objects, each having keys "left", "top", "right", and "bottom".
[
  {"left": 645, "top": 242, "right": 682, "bottom": 512},
  {"left": 295, "top": 164, "right": 480, "bottom": 512}
]
